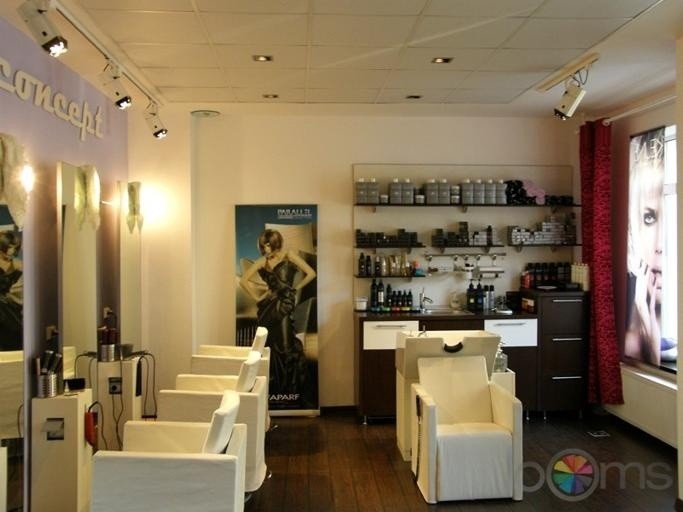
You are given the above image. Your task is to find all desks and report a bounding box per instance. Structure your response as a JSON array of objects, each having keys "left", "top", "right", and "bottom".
[{"left": 352, "top": 311, "right": 537, "bottom": 424}]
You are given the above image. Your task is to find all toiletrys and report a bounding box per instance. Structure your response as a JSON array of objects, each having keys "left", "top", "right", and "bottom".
[{"left": 450, "top": 290, "right": 461, "bottom": 307}]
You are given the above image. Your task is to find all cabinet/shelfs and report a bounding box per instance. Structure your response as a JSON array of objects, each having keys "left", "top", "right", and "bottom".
[
  {"left": 89, "top": 354, "right": 141, "bottom": 451},
  {"left": 31, "top": 391, "right": 91, "bottom": 511},
  {"left": 505, "top": 287, "right": 592, "bottom": 426}
]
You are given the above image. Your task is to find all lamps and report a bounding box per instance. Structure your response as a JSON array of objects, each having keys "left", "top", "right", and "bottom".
[
  {"left": 142, "top": 97, "right": 167, "bottom": 140},
  {"left": 16, "top": 1, "right": 69, "bottom": 60},
  {"left": 552, "top": 63, "right": 592, "bottom": 121},
  {"left": 92, "top": 58, "right": 131, "bottom": 111}
]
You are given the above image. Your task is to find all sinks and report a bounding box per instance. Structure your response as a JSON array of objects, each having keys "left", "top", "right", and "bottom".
[{"left": 422, "top": 307, "right": 474, "bottom": 316}]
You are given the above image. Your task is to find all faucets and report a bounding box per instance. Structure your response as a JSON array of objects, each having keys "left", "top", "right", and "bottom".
[{"left": 418, "top": 291, "right": 433, "bottom": 309}]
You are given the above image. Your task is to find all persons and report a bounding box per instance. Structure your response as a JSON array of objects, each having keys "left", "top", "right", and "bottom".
[
  {"left": 239, "top": 229, "right": 316, "bottom": 392},
  {"left": 626, "top": 128, "right": 663, "bottom": 366}
]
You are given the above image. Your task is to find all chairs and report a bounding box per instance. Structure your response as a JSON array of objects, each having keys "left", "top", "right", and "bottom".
[
  {"left": 411, "top": 356, "right": 524, "bottom": 504},
  {"left": 158, "top": 352, "right": 266, "bottom": 500},
  {"left": 89, "top": 390, "right": 247, "bottom": 512},
  {"left": 190, "top": 326, "right": 271, "bottom": 431},
  {"left": 393, "top": 337, "right": 515, "bottom": 462}
]
